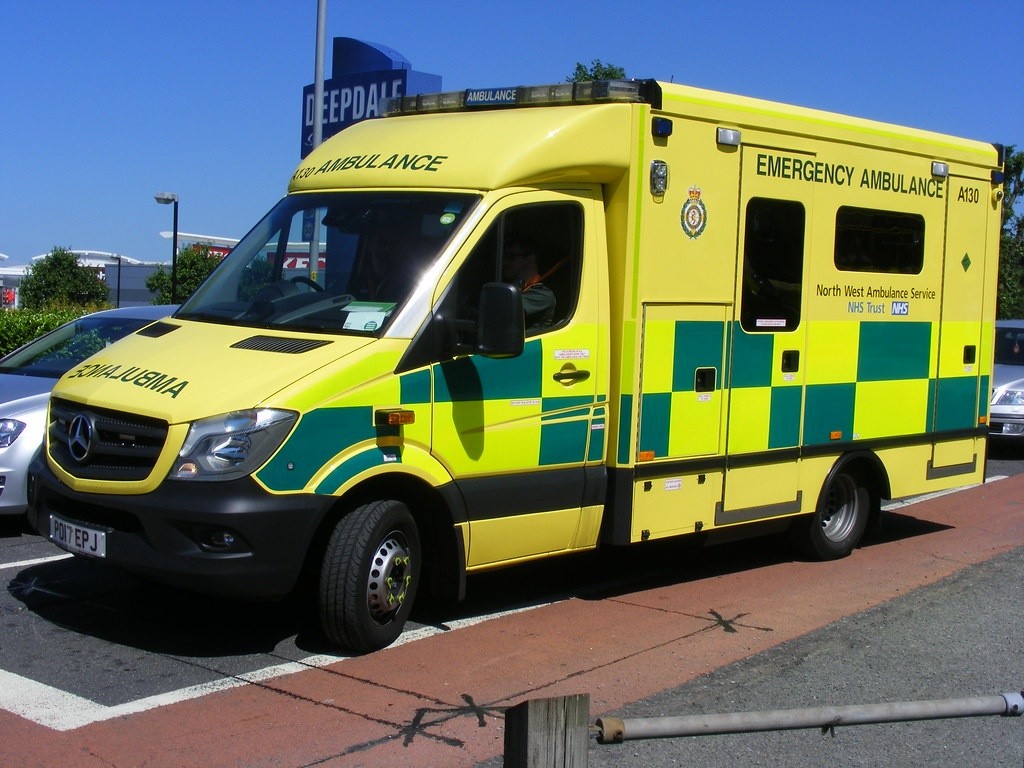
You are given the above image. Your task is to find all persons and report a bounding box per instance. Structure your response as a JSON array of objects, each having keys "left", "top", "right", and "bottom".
[{"left": 431, "top": 235, "right": 556, "bottom": 364}]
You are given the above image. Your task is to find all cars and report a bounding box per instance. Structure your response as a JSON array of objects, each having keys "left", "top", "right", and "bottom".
[
  {"left": 989, "top": 320, "right": 1024, "bottom": 457},
  {"left": 0, "top": 304, "right": 182, "bottom": 515}
]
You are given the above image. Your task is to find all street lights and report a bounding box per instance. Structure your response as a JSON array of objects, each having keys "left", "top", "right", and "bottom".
[
  {"left": 154, "top": 193, "right": 179, "bottom": 305},
  {"left": 110, "top": 253, "right": 121, "bottom": 307}
]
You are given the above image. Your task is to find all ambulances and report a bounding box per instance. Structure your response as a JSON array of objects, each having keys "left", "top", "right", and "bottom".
[{"left": 23, "top": 78, "right": 1005, "bottom": 649}]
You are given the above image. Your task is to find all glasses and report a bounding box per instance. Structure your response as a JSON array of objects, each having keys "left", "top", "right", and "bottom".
[{"left": 503, "top": 251, "right": 533, "bottom": 260}]
